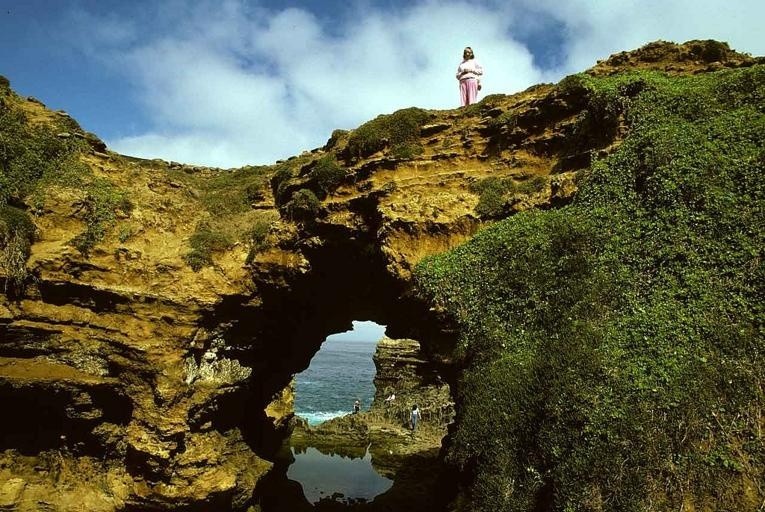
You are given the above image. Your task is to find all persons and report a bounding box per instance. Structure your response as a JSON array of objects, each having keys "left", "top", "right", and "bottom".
[
  {"left": 408, "top": 405, "right": 423, "bottom": 431},
  {"left": 384, "top": 390, "right": 396, "bottom": 404},
  {"left": 456, "top": 47, "right": 483, "bottom": 107},
  {"left": 353, "top": 400, "right": 360, "bottom": 414}
]
